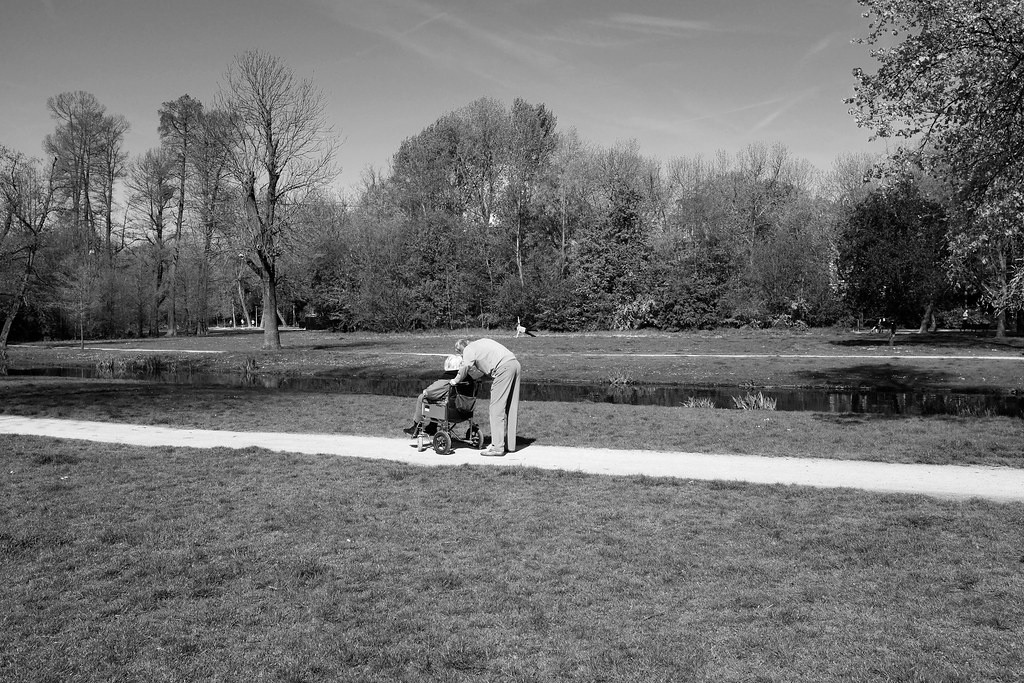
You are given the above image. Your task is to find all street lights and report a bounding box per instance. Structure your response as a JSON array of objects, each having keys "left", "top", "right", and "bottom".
[
  {"left": 238, "top": 253, "right": 245, "bottom": 327},
  {"left": 88, "top": 250, "right": 95, "bottom": 339}
]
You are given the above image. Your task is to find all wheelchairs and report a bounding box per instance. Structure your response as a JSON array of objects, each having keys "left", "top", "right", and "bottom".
[{"left": 403, "top": 380, "right": 485, "bottom": 455}]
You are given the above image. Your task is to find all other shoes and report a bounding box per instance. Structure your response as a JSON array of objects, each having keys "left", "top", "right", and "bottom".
[
  {"left": 504, "top": 445, "right": 516, "bottom": 453},
  {"left": 424, "top": 427, "right": 437, "bottom": 434},
  {"left": 480, "top": 448, "right": 504, "bottom": 456},
  {"left": 402, "top": 427, "right": 420, "bottom": 436}
]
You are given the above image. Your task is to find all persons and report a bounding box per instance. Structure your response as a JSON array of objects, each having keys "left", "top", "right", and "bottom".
[
  {"left": 515, "top": 316, "right": 537, "bottom": 338},
  {"left": 449, "top": 339, "right": 521, "bottom": 456},
  {"left": 960, "top": 309, "right": 968, "bottom": 331},
  {"left": 402, "top": 355, "right": 463, "bottom": 438}
]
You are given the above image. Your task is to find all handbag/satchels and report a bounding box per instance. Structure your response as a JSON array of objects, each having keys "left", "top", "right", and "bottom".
[{"left": 455, "top": 395, "right": 476, "bottom": 413}]
